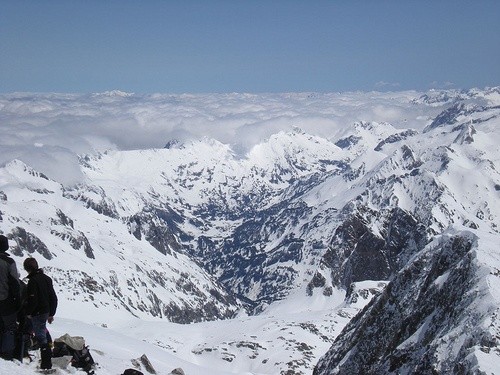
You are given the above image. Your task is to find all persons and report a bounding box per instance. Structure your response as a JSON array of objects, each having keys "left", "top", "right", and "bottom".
[
  {"left": 0, "top": 234, "right": 24, "bottom": 362},
  {"left": 19, "top": 257, "right": 58, "bottom": 372}
]
